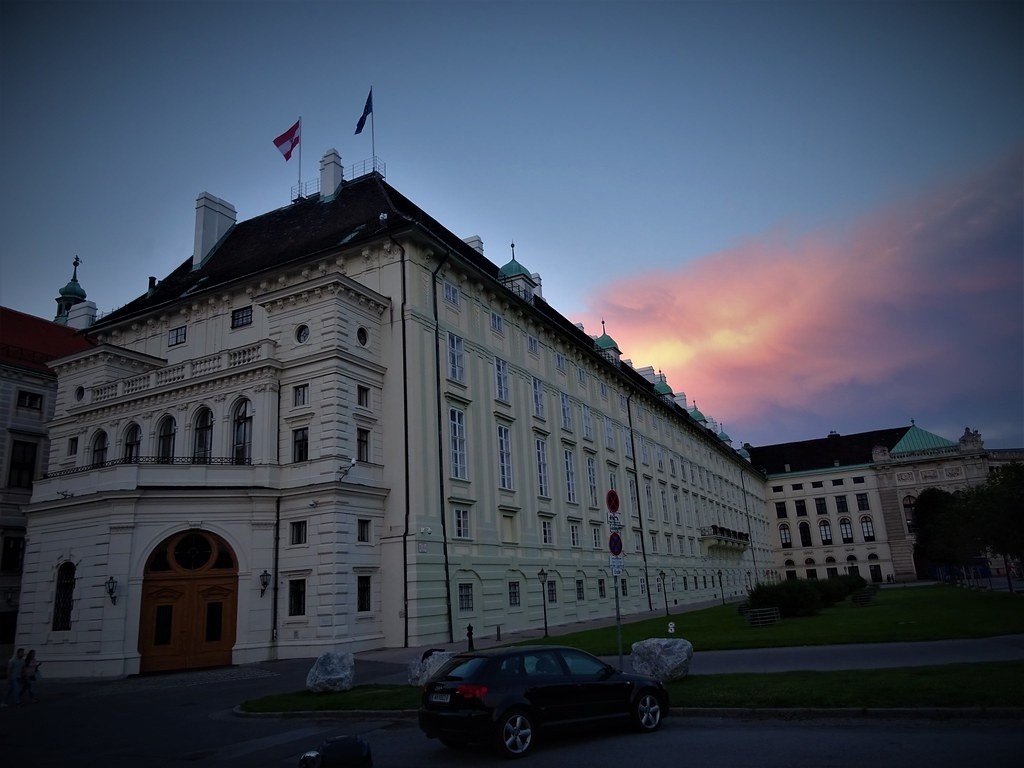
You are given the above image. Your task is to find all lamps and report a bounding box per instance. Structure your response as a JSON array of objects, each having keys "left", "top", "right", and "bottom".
[
  {"left": 105, "top": 576, "right": 118, "bottom": 605},
  {"left": 259, "top": 570, "right": 271, "bottom": 597}
]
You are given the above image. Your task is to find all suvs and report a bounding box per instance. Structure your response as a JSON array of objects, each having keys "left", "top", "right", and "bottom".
[{"left": 417, "top": 645, "right": 671, "bottom": 760}]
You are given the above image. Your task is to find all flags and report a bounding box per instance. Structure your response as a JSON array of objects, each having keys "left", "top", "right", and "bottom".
[
  {"left": 355, "top": 89, "right": 372, "bottom": 135},
  {"left": 273, "top": 121, "right": 299, "bottom": 162}
]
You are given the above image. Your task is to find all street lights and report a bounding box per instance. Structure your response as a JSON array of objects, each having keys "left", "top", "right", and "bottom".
[
  {"left": 659, "top": 570, "right": 669, "bottom": 616},
  {"left": 537, "top": 567, "right": 551, "bottom": 639},
  {"left": 717, "top": 568, "right": 726, "bottom": 606},
  {"left": 746, "top": 570, "right": 754, "bottom": 592}
]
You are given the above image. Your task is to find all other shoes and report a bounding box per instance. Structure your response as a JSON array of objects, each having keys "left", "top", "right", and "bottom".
[
  {"left": 1, "top": 704, "right": 8, "bottom": 708},
  {"left": 32, "top": 700, "right": 38, "bottom": 704},
  {"left": 16, "top": 702, "right": 25, "bottom": 706}
]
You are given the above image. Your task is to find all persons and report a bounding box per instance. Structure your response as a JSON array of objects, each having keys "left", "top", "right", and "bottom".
[
  {"left": 886, "top": 574, "right": 895, "bottom": 583},
  {"left": 1007, "top": 559, "right": 1018, "bottom": 578}
]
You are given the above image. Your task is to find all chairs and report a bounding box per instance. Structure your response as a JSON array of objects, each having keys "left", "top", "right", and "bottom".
[
  {"left": 506, "top": 657, "right": 519, "bottom": 673},
  {"left": 535, "top": 658, "right": 555, "bottom": 673}
]
